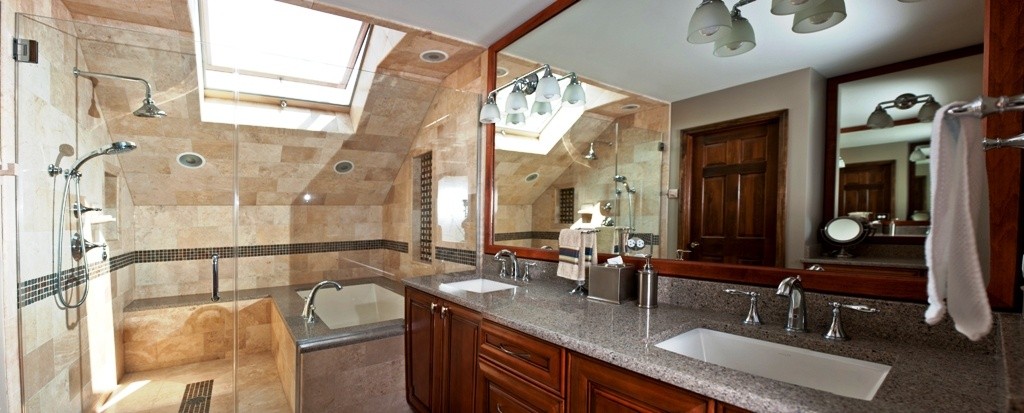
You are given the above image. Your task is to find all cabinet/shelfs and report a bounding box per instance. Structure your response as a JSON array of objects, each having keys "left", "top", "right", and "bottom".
[
  {"left": 565, "top": 350, "right": 765, "bottom": 413},
  {"left": 399, "top": 286, "right": 480, "bottom": 413},
  {"left": 478, "top": 320, "right": 569, "bottom": 413}
]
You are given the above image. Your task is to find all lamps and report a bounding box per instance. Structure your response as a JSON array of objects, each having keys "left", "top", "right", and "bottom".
[
  {"left": 504, "top": 71, "right": 588, "bottom": 127},
  {"left": 909, "top": 145, "right": 934, "bottom": 162},
  {"left": 478, "top": 63, "right": 559, "bottom": 123},
  {"left": 684, "top": 0, "right": 821, "bottom": 45},
  {"left": 712, "top": 0, "right": 846, "bottom": 58},
  {"left": 866, "top": 92, "right": 944, "bottom": 129}
]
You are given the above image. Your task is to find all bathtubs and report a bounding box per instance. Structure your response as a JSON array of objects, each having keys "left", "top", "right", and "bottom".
[{"left": 296, "top": 281, "right": 405, "bottom": 331}]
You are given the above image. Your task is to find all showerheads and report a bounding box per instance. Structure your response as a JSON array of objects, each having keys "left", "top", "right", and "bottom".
[
  {"left": 585, "top": 140, "right": 616, "bottom": 162},
  {"left": 613, "top": 175, "right": 635, "bottom": 233},
  {"left": 74, "top": 67, "right": 164, "bottom": 120},
  {"left": 56, "top": 140, "right": 139, "bottom": 307}
]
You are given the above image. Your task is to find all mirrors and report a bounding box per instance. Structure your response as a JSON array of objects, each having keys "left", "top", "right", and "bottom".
[
  {"left": 482, "top": 1, "right": 1024, "bottom": 309},
  {"left": 821, "top": 43, "right": 984, "bottom": 244},
  {"left": 823, "top": 216, "right": 871, "bottom": 258},
  {"left": 906, "top": 141, "right": 935, "bottom": 221}
]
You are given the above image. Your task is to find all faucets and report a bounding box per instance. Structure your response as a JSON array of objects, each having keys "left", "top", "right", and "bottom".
[
  {"left": 301, "top": 280, "right": 344, "bottom": 323},
  {"left": 493, "top": 249, "right": 521, "bottom": 281},
  {"left": 774, "top": 275, "right": 808, "bottom": 332}
]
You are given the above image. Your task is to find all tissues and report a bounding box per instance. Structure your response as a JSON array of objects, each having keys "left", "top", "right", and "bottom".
[{"left": 587, "top": 255, "right": 636, "bottom": 305}]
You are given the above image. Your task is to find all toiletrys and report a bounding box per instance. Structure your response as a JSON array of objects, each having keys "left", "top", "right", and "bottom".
[{"left": 637, "top": 254, "right": 658, "bottom": 308}]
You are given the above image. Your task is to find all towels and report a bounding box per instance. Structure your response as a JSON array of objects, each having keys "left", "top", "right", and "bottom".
[
  {"left": 556, "top": 228, "right": 599, "bottom": 282},
  {"left": 924, "top": 100, "right": 995, "bottom": 343},
  {"left": 594, "top": 227, "right": 628, "bottom": 256}
]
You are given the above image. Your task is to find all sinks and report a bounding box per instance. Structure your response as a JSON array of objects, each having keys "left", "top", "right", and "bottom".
[
  {"left": 650, "top": 318, "right": 894, "bottom": 401},
  {"left": 446, "top": 278, "right": 519, "bottom": 294}
]
